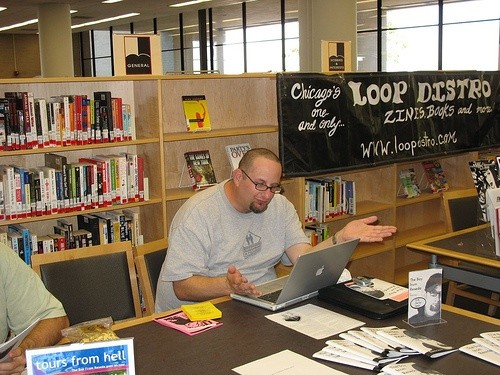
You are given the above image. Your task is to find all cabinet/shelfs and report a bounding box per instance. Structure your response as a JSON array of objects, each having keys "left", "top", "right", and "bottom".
[{"left": 1, "top": 71, "right": 500, "bottom": 326}]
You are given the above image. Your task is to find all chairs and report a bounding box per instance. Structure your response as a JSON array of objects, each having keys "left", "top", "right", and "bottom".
[
  {"left": 443, "top": 188, "right": 499, "bottom": 316},
  {"left": 30, "top": 240, "right": 142, "bottom": 327}
]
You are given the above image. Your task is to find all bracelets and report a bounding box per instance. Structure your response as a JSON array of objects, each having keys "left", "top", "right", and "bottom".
[{"left": 332, "top": 233, "right": 336, "bottom": 245}]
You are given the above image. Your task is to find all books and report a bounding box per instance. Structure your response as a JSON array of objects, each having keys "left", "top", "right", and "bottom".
[
  {"left": 313, "top": 326, "right": 500, "bottom": 375},
  {"left": 0, "top": 91, "right": 133, "bottom": 151},
  {"left": 182, "top": 95, "right": 211, "bottom": 132},
  {"left": 181, "top": 300, "right": 222, "bottom": 321},
  {"left": 422, "top": 161, "right": 449, "bottom": 193},
  {"left": 305, "top": 175, "right": 356, "bottom": 247},
  {"left": 0, "top": 209, "right": 143, "bottom": 265},
  {"left": 225, "top": 143, "right": 252, "bottom": 170},
  {"left": 0, "top": 153, "right": 149, "bottom": 220},
  {"left": 184, "top": 150, "right": 217, "bottom": 190},
  {"left": 399, "top": 168, "right": 421, "bottom": 199},
  {"left": 469, "top": 155, "right": 500, "bottom": 256}
]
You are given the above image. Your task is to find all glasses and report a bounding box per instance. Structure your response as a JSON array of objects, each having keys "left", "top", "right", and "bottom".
[
  {"left": 242, "top": 170, "right": 284, "bottom": 194},
  {"left": 425, "top": 289, "right": 440, "bottom": 297}
]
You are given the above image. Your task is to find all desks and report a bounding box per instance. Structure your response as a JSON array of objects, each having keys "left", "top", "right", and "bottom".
[
  {"left": 24, "top": 273, "right": 500, "bottom": 375},
  {"left": 406, "top": 223, "right": 500, "bottom": 316}
]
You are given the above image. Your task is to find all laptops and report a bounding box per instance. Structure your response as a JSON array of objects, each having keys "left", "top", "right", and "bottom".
[{"left": 230, "top": 238, "right": 361, "bottom": 311}]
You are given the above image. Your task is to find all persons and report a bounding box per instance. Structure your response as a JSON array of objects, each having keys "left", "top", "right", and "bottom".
[
  {"left": 0, "top": 242, "right": 69, "bottom": 375},
  {"left": 408, "top": 273, "right": 442, "bottom": 325},
  {"left": 403, "top": 331, "right": 456, "bottom": 351},
  {"left": 155, "top": 148, "right": 398, "bottom": 313}
]
